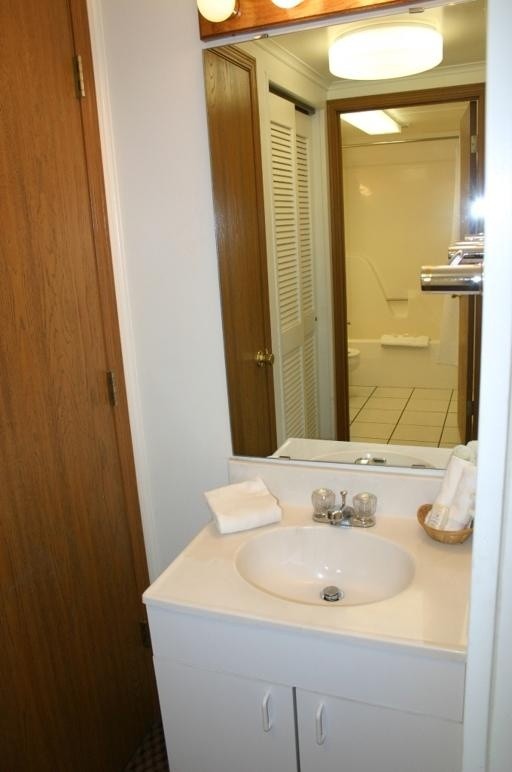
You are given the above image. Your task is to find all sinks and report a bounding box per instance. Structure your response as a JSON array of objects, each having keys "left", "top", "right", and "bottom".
[
  {"left": 305, "top": 449, "right": 439, "bottom": 469},
  {"left": 227, "top": 515, "right": 422, "bottom": 615}
]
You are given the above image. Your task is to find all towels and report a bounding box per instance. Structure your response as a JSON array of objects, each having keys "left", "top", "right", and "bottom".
[
  {"left": 423, "top": 455, "right": 478, "bottom": 532},
  {"left": 379, "top": 333, "right": 430, "bottom": 349},
  {"left": 204, "top": 477, "right": 283, "bottom": 534}
]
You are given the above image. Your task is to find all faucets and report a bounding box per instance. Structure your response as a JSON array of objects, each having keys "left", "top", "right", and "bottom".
[
  {"left": 352, "top": 455, "right": 389, "bottom": 466},
  {"left": 312, "top": 487, "right": 378, "bottom": 527}
]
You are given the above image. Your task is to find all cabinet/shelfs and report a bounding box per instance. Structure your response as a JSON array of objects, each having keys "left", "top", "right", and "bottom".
[
  {"left": 268, "top": 90, "right": 319, "bottom": 444},
  {"left": 146, "top": 606, "right": 465, "bottom": 772}
]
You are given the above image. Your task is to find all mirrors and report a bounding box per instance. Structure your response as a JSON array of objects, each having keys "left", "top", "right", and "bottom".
[{"left": 202, "top": 0, "right": 492, "bottom": 476}]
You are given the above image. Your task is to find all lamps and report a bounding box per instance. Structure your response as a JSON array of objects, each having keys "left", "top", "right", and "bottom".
[
  {"left": 327, "top": 22, "right": 444, "bottom": 80},
  {"left": 197, "top": 0, "right": 240, "bottom": 22},
  {"left": 341, "top": 108, "right": 402, "bottom": 137}
]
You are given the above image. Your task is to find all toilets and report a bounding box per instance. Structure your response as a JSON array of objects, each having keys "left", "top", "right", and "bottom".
[{"left": 348, "top": 345, "right": 361, "bottom": 373}]
